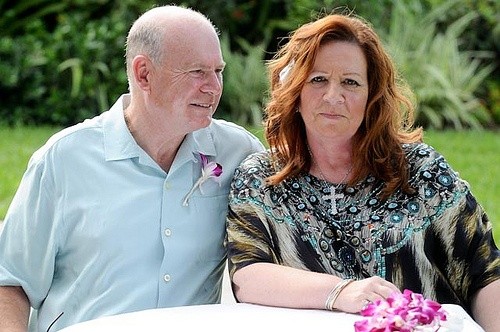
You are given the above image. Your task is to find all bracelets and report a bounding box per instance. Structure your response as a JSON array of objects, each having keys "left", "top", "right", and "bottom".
[{"left": 326, "top": 277, "right": 355, "bottom": 311}]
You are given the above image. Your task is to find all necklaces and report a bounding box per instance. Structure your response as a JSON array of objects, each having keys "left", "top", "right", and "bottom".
[{"left": 307, "top": 145, "right": 357, "bottom": 214}]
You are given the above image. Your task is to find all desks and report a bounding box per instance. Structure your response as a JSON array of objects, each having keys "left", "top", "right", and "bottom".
[{"left": 63, "top": 303, "right": 486, "bottom": 332}]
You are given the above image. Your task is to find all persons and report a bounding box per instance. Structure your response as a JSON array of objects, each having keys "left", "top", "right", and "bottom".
[
  {"left": 224, "top": 15, "right": 500, "bottom": 332},
  {"left": 0, "top": 6, "right": 267, "bottom": 332}
]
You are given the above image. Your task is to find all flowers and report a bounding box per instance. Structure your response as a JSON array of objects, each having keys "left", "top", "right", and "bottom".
[
  {"left": 182, "top": 153, "right": 223, "bottom": 206},
  {"left": 355, "top": 289, "right": 449, "bottom": 332}
]
